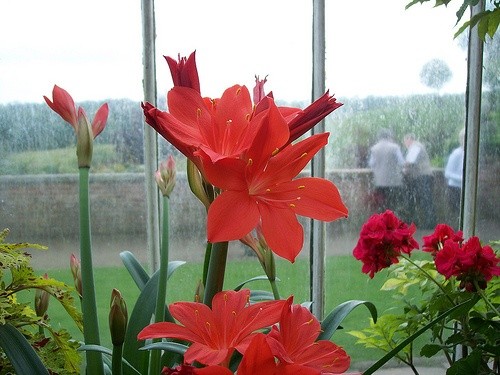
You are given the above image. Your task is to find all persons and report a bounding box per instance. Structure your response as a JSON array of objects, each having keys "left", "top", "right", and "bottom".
[
  {"left": 400, "top": 133, "right": 439, "bottom": 231},
  {"left": 444, "top": 126, "right": 467, "bottom": 231},
  {"left": 369, "top": 128, "right": 407, "bottom": 217}
]
